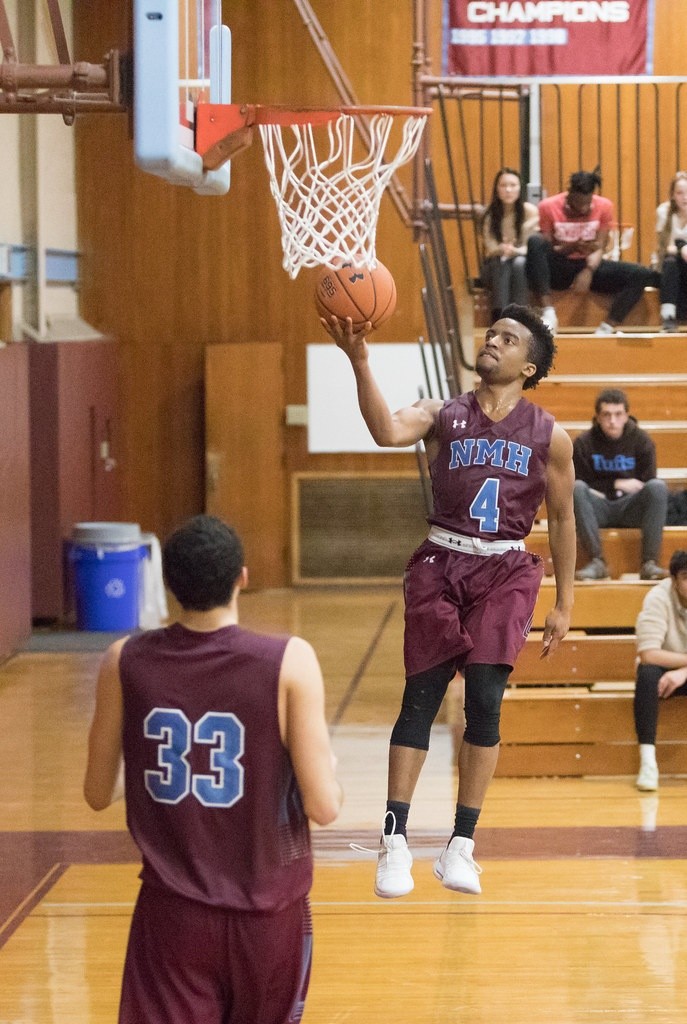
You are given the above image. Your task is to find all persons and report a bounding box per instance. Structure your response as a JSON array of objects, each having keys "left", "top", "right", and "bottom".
[
  {"left": 320, "top": 304, "right": 577, "bottom": 898},
  {"left": 478, "top": 168, "right": 687, "bottom": 794},
  {"left": 85, "top": 515, "right": 346, "bottom": 1024}
]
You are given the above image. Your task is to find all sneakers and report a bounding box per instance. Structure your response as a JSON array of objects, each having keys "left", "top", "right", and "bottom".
[
  {"left": 575, "top": 557, "right": 611, "bottom": 581},
  {"left": 640, "top": 560, "right": 668, "bottom": 580},
  {"left": 349, "top": 811, "right": 414, "bottom": 897},
  {"left": 433, "top": 836, "right": 482, "bottom": 894}
]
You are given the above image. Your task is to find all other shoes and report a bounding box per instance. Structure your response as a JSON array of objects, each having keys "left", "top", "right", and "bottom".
[
  {"left": 539, "top": 316, "right": 556, "bottom": 334},
  {"left": 639, "top": 799, "right": 660, "bottom": 832},
  {"left": 637, "top": 761, "right": 658, "bottom": 790},
  {"left": 660, "top": 318, "right": 678, "bottom": 332},
  {"left": 594, "top": 321, "right": 621, "bottom": 334}
]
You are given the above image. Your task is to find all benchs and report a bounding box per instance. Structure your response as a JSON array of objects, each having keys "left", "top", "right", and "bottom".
[{"left": 470, "top": 287, "right": 686, "bottom": 777}]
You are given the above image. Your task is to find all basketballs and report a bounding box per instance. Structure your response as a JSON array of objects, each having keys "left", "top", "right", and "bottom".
[{"left": 313, "top": 253, "right": 397, "bottom": 335}]
